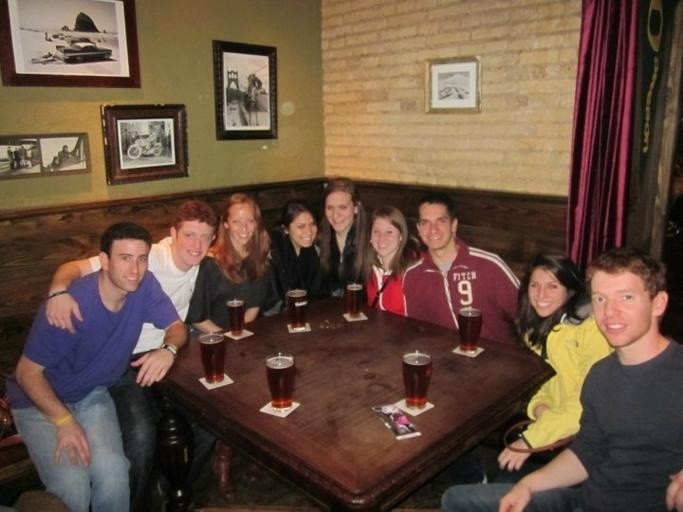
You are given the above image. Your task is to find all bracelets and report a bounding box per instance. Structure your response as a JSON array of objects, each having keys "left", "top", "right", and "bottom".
[
  {"left": 518, "top": 431, "right": 534, "bottom": 450},
  {"left": 159, "top": 343, "right": 178, "bottom": 357},
  {"left": 54, "top": 414, "right": 73, "bottom": 426},
  {"left": 46, "top": 290, "right": 70, "bottom": 301}
]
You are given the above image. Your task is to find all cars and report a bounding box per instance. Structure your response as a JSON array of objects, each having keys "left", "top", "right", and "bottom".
[
  {"left": 70, "top": 38, "right": 95, "bottom": 46},
  {"left": 51, "top": 43, "right": 112, "bottom": 61}
]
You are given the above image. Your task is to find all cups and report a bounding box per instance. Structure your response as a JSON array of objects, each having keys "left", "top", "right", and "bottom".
[
  {"left": 346, "top": 285, "right": 362, "bottom": 318},
  {"left": 403, "top": 354, "right": 431, "bottom": 410},
  {"left": 227, "top": 301, "right": 243, "bottom": 336},
  {"left": 198, "top": 333, "right": 226, "bottom": 383},
  {"left": 458, "top": 309, "right": 480, "bottom": 353},
  {"left": 265, "top": 355, "right": 295, "bottom": 412},
  {"left": 286, "top": 290, "right": 307, "bottom": 331}
]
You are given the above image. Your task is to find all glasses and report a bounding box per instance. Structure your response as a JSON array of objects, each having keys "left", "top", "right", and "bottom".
[{"left": 179, "top": 229, "right": 216, "bottom": 243}]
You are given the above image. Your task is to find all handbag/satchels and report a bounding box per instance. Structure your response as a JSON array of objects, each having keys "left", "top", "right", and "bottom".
[{"left": 497, "top": 415, "right": 570, "bottom": 462}]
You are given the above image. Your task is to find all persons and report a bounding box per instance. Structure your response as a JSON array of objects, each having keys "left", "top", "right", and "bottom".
[
  {"left": 43, "top": 199, "right": 220, "bottom": 511},
  {"left": 360, "top": 202, "right": 426, "bottom": 316},
  {"left": 266, "top": 199, "right": 323, "bottom": 312},
  {"left": 184, "top": 192, "right": 280, "bottom": 340},
  {"left": 440, "top": 247, "right": 682, "bottom": 512},
  {"left": 6, "top": 146, "right": 33, "bottom": 169},
  {"left": 247, "top": 79, "right": 259, "bottom": 111},
  {"left": 401, "top": 197, "right": 522, "bottom": 345},
  {"left": 317, "top": 176, "right": 370, "bottom": 305},
  {"left": 40, "top": 32, "right": 68, "bottom": 59},
  {"left": 7, "top": 221, "right": 189, "bottom": 512},
  {"left": 469, "top": 252, "right": 615, "bottom": 483},
  {"left": 146, "top": 125, "right": 158, "bottom": 152}
]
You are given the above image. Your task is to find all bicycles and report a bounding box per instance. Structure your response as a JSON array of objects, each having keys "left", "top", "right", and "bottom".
[{"left": 128, "top": 136, "right": 161, "bottom": 158}]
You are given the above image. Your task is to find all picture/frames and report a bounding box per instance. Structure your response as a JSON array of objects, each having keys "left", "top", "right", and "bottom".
[
  {"left": 0, "top": 129, "right": 93, "bottom": 183},
  {"left": 209, "top": 37, "right": 280, "bottom": 143},
  {"left": 423, "top": 56, "right": 484, "bottom": 117},
  {"left": 0, "top": 0, "right": 142, "bottom": 90},
  {"left": 97, "top": 102, "right": 192, "bottom": 186}
]
